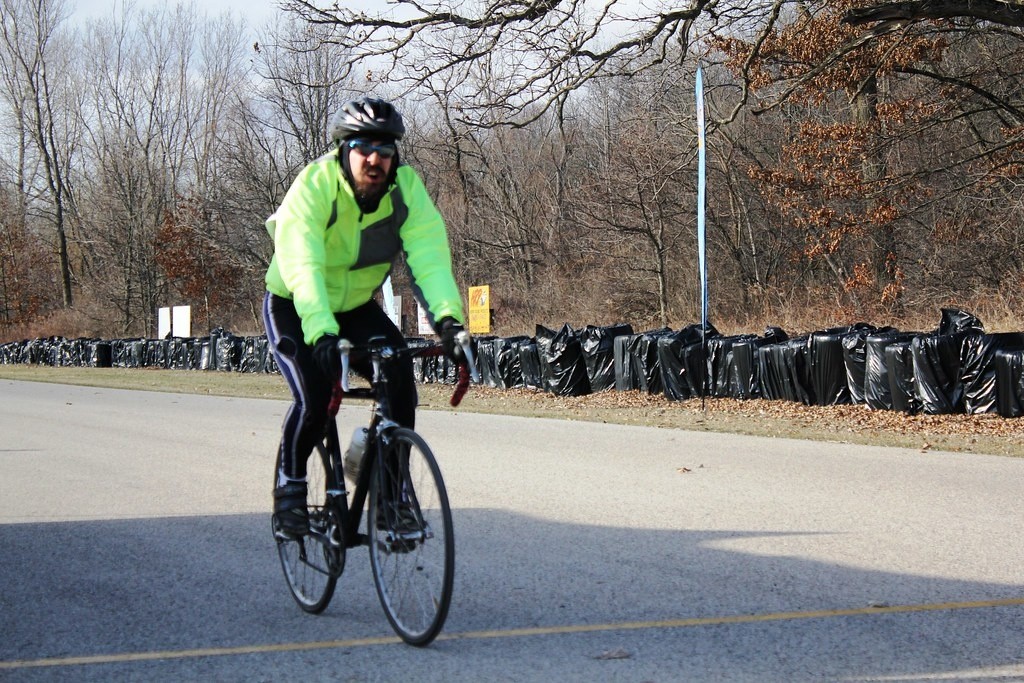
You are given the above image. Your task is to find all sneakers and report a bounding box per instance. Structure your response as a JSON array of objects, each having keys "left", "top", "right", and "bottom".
[
  {"left": 272, "top": 483, "right": 311, "bottom": 534},
  {"left": 371, "top": 501, "right": 433, "bottom": 544}
]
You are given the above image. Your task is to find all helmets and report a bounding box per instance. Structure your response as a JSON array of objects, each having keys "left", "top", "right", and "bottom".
[{"left": 330, "top": 96, "right": 404, "bottom": 150}]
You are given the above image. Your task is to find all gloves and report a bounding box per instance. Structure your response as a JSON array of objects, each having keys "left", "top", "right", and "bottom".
[
  {"left": 441, "top": 318, "right": 478, "bottom": 366},
  {"left": 312, "top": 334, "right": 342, "bottom": 385}
]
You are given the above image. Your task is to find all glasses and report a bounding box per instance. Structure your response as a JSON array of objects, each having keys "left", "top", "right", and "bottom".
[{"left": 344, "top": 139, "right": 397, "bottom": 159}]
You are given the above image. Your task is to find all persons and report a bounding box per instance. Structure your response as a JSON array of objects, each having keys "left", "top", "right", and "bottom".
[{"left": 261, "top": 96, "right": 478, "bottom": 538}]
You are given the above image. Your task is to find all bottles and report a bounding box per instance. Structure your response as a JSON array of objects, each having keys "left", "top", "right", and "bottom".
[{"left": 344, "top": 426, "right": 369, "bottom": 482}]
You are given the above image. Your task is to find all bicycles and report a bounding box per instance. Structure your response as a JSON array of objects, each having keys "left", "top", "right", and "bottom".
[{"left": 271, "top": 330, "right": 483, "bottom": 647}]
